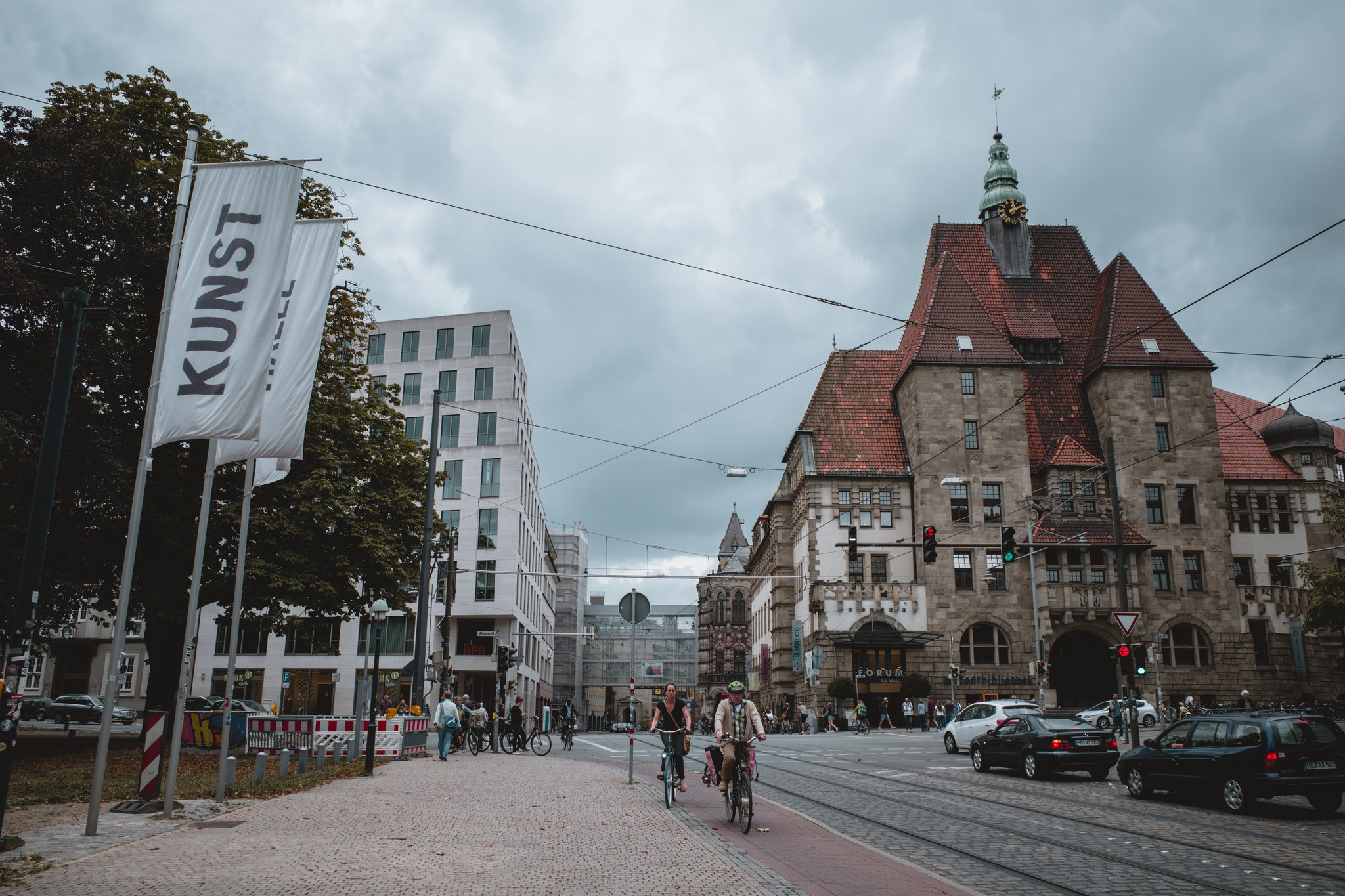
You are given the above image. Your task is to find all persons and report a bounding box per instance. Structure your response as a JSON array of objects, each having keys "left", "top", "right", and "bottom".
[
  {"left": 1184, "top": 693, "right": 1194, "bottom": 713},
  {"left": 878, "top": 697, "right": 897, "bottom": 731},
  {"left": 779, "top": 703, "right": 794, "bottom": 729},
  {"left": 433, "top": 690, "right": 461, "bottom": 762},
  {"left": 558, "top": 697, "right": 577, "bottom": 746},
  {"left": 395, "top": 699, "right": 410, "bottom": 736},
  {"left": 451, "top": 694, "right": 505, "bottom": 753},
  {"left": 714, "top": 681, "right": 767, "bottom": 817},
  {"left": 1029, "top": 694, "right": 1038, "bottom": 706},
  {"left": 852, "top": 700, "right": 867, "bottom": 732},
  {"left": 902, "top": 696, "right": 955, "bottom": 733},
  {"left": 649, "top": 681, "right": 692, "bottom": 792},
  {"left": 796, "top": 702, "right": 808, "bottom": 734},
  {"left": 634, "top": 722, "right": 640, "bottom": 733},
  {"left": 379, "top": 693, "right": 392, "bottom": 716},
  {"left": 699, "top": 713, "right": 715, "bottom": 736},
  {"left": 511, "top": 696, "right": 531, "bottom": 755},
  {"left": 821, "top": 702, "right": 838, "bottom": 733},
  {"left": 1171, "top": 743, "right": 1184, "bottom": 748},
  {"left": 422, "top": 696, "right": 432, "bottom": 736},
  {"left": 1108, "top": 693, "right": 1126, "bottom": 741},
  {"left": 760, "top": 706, "right": 773, "bottom": 731},
  {"left": 551, "top": 716, "right": 578, "bottom": 734},
  {"left": 1237, "top": 689, "right": 1254, "bottom": 713}
]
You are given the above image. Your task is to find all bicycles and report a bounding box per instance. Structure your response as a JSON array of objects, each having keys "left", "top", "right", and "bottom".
[
  {"left": 714, "top": 732, "right": 762, "bottom": 834},
  {"left": 684, "top": 715, "right": 811, "bottom": 735},
  {"left": 651, "top": 726, "right": 687, "bottom": 809},
  {"left": 559, "top": 715, "right": 578, "bottom": 751},
  {"left": 851, "top": 713, "right": 870, "bottom": 735},
  {"left": 448, "top": 713, "right": 552, "bottom": 756}
]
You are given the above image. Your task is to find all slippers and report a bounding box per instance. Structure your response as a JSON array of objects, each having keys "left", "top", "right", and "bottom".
[
  {"left": 657, "top": 772, "right": 665, "bottom": 778},
  {"left": 680, "top": 781, "right": 687, "bottom": 792}
]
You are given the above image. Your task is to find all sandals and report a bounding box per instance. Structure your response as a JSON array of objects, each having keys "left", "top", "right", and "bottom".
[{"left": 453, "top": 746, "right": 458, "bottom": 753}]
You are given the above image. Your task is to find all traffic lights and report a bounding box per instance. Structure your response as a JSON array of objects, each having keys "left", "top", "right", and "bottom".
[
  {"left": 957, "top": 666, "right": 966, "bottom": 685},
  {"left": 497, "top": 645, "right": 509, "bottom": 673},
  {"left": 507, "top": 680, "right": 514, "bottom": 690},
  {"left": 508, "top": 646, "right": 517, "bottom": 668},
  {"left": 1122, "top": 685, "right": 1128, "bottom": 701},
  {"left": 1037, "top": 660, "right": 1052, "bottom": 681},
  {"left": 922, "top": 526, "right": 938, "bottom": 562},
  {"left": 1109, "top": 645, "right": 1147, "bottom": 677},
  {"left": 510, "top": 666, "right": 519, "bottom": 683},
  {"left": 951, "top": 667, "right": 958, "bottom": 679},
  {"left": 1000, "top": 526, "right": 1017, "bottom": 563},
  {"left": 510, "top": 680, "right": 518, "bottom": 697},
  {"left": 848, "top": 526, "right": 858, "bottom": 561}
]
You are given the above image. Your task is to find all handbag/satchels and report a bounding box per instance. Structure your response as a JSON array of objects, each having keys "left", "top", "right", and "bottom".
[
  {"left": 704, "top": 744, "right": 724, "bottom": 787},
  {"left": 1188, "top": 701, "right": 1191, "bottom": 707},
  {"left": 782, "top": 713, "right": 786, "bottom": 719},
  {"left": 749, "top": 746, "right": 756, "bottom": 780},
  {"left": 682, "top": 737, "right": 691, "bottom": 756},
  {"left": 442, "top": 716, "right": 458, "bottom": 732}
]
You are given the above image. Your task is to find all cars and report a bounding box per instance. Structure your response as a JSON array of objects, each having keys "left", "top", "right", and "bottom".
[
  {"left": 943, "top": 698, "right": 1342, "bottom": 781},
  {"left": 185, "top": 695, "right": 271, "bottom": 715},
  {"left": 608, "top": 722, "right": 635, "bottom": 734}
]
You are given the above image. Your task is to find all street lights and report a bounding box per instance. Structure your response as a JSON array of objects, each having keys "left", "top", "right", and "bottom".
[
  {"left": 364, "top": 599, "right": 390, "bottom": 777},
  {"left": 980, "top": 519, "right": 1089, "bottom": 711}
]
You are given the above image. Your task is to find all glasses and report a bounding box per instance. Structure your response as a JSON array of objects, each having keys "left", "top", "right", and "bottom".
[{"left": 731, "top": 691, "right": 743, "bottom": 696}]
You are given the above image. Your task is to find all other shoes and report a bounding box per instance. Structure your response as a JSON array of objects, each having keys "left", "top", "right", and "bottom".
[
  {"left": 718, "top": 780, "right": 728, "bottom": 790},
  {"left": 744, "top": 808, "right": 754, "bottom": 817},
  {"left": 878, "top": 726, "right": 897, "bottom": 730},
  {"left": 512, "top": 747, "right": 531, "bottom": 755},
  {"left": 491, "top": 749, "right": 503, "bottom": 753},
  {"left": 571, "top": 742, "right": 574, "bottom": 746},
  {"left": 1119, "top": 736, "right": 1126, "bottom": 741},
  {"left": 439, "top": 755, "right": 448, "bottom": 762},
  {"left": 906, "top": 728, "right": 944, "bottom": 733},
  {"left": 801, "top": 727, "right": 838, "bottom": 734}
]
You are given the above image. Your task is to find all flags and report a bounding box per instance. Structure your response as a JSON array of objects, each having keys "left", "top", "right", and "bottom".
[
  {"left": 214, "top": 221, "right": 343, "bottom": 487},
  {"left": 151, "top": 161, "right": 306, "bottom": 450}
]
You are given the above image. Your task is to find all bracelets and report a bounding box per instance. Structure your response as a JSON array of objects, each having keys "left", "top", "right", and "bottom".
[{"left": 434, "top": 723, "right": 437, "bottom": 726}]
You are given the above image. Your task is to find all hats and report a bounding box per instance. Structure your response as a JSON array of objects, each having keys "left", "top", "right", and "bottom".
[{"left": 884, "top": 697, "right": 888, "bottom": 700}]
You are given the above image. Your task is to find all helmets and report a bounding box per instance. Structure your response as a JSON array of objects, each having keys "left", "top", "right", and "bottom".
[{"left": 727, "top": 681, "right": 744, "bottom": 693}]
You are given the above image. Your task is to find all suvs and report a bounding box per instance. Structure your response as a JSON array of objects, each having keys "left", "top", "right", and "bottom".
[
  {"left": 1115, "top": 708, "right": 1344, "bottom": 815},
  {"left": 19, "top": 694, "right": 138, "bottom": 726}
]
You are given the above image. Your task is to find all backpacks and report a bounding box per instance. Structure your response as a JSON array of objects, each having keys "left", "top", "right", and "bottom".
[
  {"left": 860, "top": 704, "right": 867, "bottom": 713},
  {"left": 878, "top": 704, "right": 881, "bottom": 711},
  {"left": 1108, "top": 701, "right": 1122, "bottom": 718},
  {"left": 765, "top": 710, "right": 773, "bottom": 721},
  {"left": 821, "top": 705, "right": 836, "bottom": 716}
]
